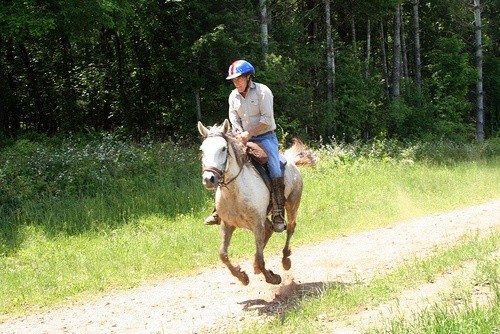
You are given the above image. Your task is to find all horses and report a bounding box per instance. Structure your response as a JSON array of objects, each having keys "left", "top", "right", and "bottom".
[{"left": 196, "top": 118, "right": 318, "bottom": 286}]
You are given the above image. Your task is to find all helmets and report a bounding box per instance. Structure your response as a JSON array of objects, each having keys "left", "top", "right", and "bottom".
[{"left": 225, "top": 59, "right": 255, "bottom": 80}]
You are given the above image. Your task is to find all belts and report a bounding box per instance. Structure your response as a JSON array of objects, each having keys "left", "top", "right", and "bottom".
[{"left": 252, "top": 131, "right": 272, "bottom": 138}]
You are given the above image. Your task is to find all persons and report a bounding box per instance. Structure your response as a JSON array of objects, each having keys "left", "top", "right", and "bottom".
[{"left": 204, "top": 60, "right": 285, "bottom": 232}]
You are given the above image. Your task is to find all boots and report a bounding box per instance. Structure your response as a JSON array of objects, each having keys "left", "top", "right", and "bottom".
[
  {"left": 205, "top": 207, "right": 222, "bottom": 226},
  {"left": 271, "top": 178, "right": 286, "bottom": 233}
]
